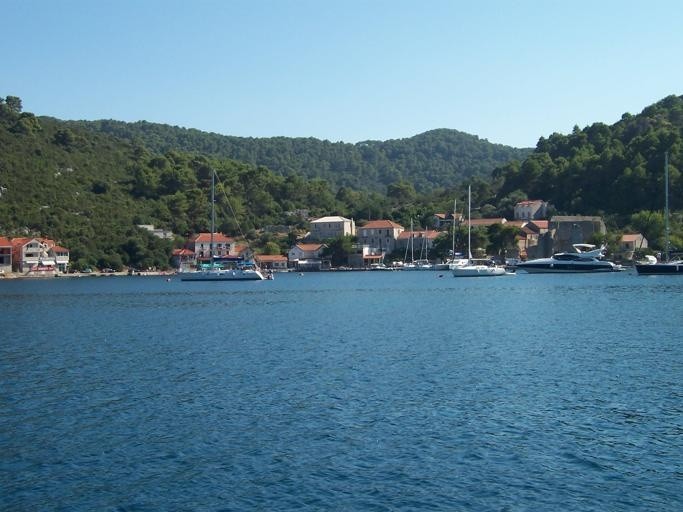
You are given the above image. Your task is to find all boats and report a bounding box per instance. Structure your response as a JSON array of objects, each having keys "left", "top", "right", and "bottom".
[
  {"left": 450, "top": 258, "right": 506, "bottom": 276},
  {"left": 514, "top": 245, "right": 622, "bottom": 272}
]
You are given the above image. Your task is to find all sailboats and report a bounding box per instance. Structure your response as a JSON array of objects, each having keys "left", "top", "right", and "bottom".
[
  {"left": 177, "top": 169, "right": 264, "bottom": 280},
  {"left": 394, "top": 184, "right": 472, "bottom": 271},
  {"left": 635, "top": 153, "right": 682, "bottom": 276}
]
